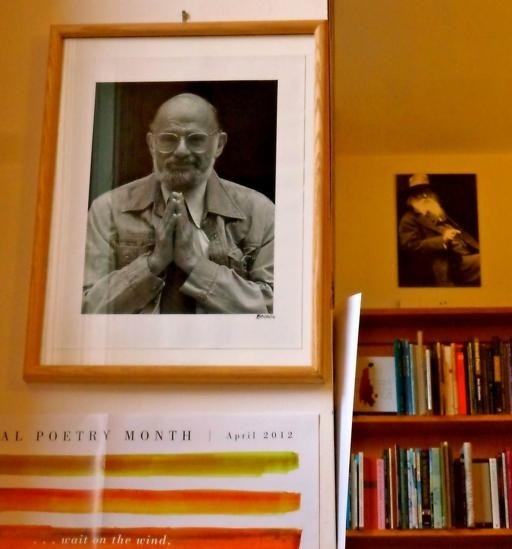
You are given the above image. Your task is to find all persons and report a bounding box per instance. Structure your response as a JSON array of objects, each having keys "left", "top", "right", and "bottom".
[
  {"left": 79, "top": 89, "right": 276, "bottom": 317},
  {"left": 396, "top": 170, "right": 482, "bottom": 287}
]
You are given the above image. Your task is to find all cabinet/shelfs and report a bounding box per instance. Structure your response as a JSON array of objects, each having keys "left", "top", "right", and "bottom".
[{"left": 345, "top": 306, "right": 512, "bottom": 549}]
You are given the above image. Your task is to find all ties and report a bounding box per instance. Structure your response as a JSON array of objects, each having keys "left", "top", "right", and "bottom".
[{"left": 158, "top": 259, "right": 195, "bottom": 313}]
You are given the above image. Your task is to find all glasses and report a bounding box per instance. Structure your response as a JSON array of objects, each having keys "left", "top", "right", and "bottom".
[{"left": 150, "top": 129, "right": 222, "bottom": 154}]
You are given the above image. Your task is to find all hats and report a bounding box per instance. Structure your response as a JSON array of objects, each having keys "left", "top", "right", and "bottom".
[{"left": 405, "top": 174, "right": 440, "bottom": 194}]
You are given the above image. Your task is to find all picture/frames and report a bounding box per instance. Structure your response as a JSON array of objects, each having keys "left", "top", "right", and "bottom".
[{"left": 20, "top": 19, "right": 332, "bottom": 388}]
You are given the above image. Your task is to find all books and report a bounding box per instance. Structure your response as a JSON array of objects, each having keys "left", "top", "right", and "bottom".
[{"left": 346, "top": 325, "right": 512, "bottom": 532}]
